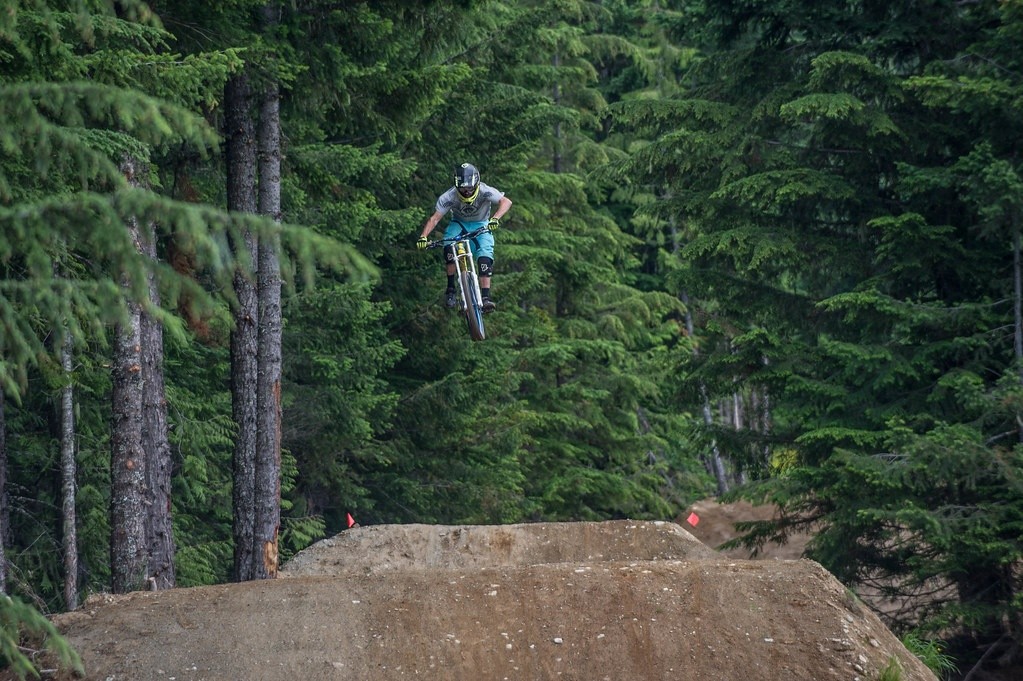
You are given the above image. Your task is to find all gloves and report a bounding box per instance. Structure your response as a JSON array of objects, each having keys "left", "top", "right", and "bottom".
[
  {"left": 416, "top": 236, "right": 427, "bottom": 248},
  {"left": 488, "top": 218, "right": 499, "bottom": 231}
]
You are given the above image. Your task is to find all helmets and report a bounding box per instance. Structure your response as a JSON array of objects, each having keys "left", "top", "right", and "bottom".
[{"left": 454, "top": 163, "right": 480, "bottom": 205}]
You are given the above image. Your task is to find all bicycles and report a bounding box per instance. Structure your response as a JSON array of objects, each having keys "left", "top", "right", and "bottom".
[{"left": 420, "top": 221, "right": 501, "bottom": 342}]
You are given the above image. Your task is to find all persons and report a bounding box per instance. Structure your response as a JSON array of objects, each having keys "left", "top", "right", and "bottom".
[{"left": 416, "top": 163, "right": 512, "bottom": 308}]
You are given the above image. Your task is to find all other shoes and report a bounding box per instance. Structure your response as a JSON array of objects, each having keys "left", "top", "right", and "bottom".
[
  {"left": 482, "top": 297, "right": 496, "bottom": 309},
  {"left": 445, "top": 287, "right": 457, "bottom": 307}
]
